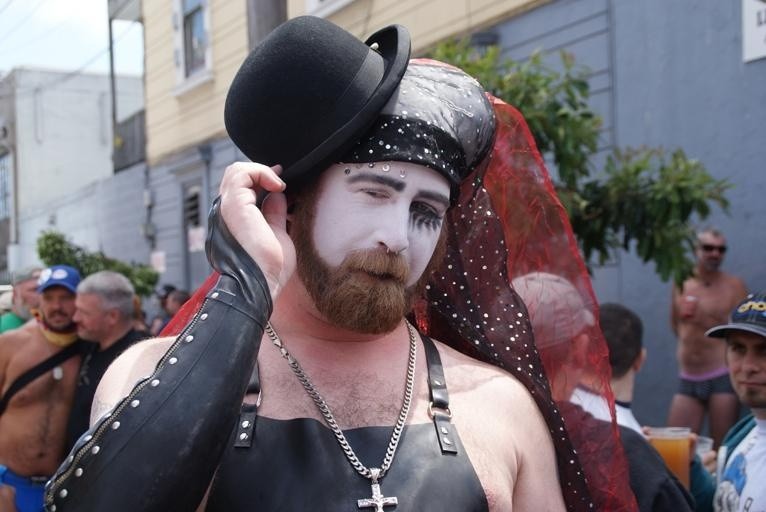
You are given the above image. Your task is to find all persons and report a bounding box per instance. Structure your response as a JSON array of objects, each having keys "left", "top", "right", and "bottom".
[
  {"left": 0, "top": 266, "right": 194, "bottom": 512},
  {"left": 640, "top": 288, "right": 766, "bottom": 512},
  {"left": 508, "top": 270, "right": 700, "bottom": 512},
  {"left": 40, "top": 15, "right": 573, "bottom": 512},
  {"left": 567, "top": 302, "right": 651, "bottom": 442},
  {"left": 666, "top": 225, "right": 748, "bottom": 461}
]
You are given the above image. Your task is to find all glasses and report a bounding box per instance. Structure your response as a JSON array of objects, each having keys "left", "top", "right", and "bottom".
[{"left": 700, "top": 243, "right": 728, "bottom": 255}]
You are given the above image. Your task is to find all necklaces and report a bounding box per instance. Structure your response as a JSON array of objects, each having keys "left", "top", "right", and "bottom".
[{"left": 262, "top": 317, "right": 419, "bottom": 511}]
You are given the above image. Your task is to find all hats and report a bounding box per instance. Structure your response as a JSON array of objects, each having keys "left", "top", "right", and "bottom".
[
  {"left": 703, "top": 290, "right": 765, "bottom": 342},
  {"left": 10, "top": 262, "right": 82, "bottom": 296},
  {"left": 224, "top": 12, "right": 414, "bottom": 186},
  {"left": 328, "top": 53, "right": 498, "bottom": 212}
]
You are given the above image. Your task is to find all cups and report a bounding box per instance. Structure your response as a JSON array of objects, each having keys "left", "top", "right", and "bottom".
[
  {"left": 644, "top": 425, "right": 691, "bottom": 493},
  {"left": 691, "top": 434, "right": 716, "bottom": 459},
  {"left": 684, "top": 294, "right": 698, "bottom": 318}
]
